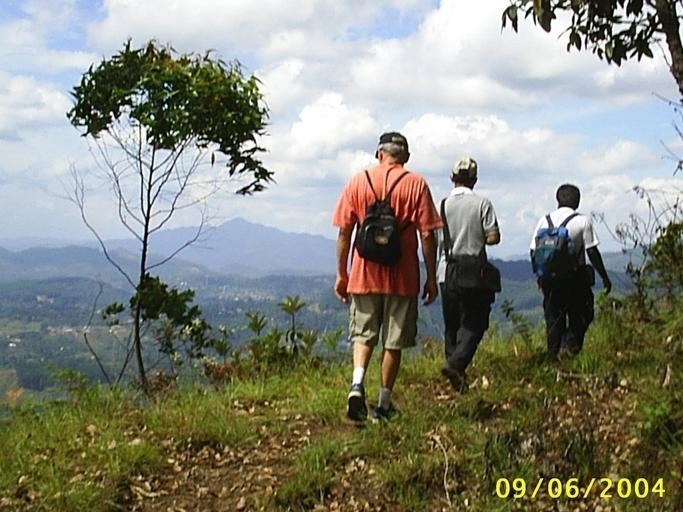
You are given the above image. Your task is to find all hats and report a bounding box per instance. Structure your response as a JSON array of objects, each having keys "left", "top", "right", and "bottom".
[
  {"left": 374, "top": 132, "right": 408, "bottom": 159},
  {"left": 452, "top": 157, "right": 478, "bottom": 177}
]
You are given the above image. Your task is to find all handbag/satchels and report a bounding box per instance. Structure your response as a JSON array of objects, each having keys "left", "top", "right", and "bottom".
[{"left": 445, "top": 254, "right": 503, "bottom": 305}]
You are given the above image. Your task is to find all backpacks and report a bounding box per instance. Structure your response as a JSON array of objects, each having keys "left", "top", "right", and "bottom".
[
  {"left": 357, "top": 170, "right": 409, "bottom": 263},
  {"left": 532, "top": 214, "right": 581, "bottom": 286}
]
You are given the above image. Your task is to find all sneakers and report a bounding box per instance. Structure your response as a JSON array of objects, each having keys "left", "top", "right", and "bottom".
[
  {"left": 547, "top": 348, "right": 576, "bottom": 363},
  {"left": 372, "top": 402, "right": 397, "bottom": 419},
  {"left": 347, "top": 382, "right": 368, "bottom": 422},
  {"left": 441, "top": 366, "right": 469, "bottom": 395}
]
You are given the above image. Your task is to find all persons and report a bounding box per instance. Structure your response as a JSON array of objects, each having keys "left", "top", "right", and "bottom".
[
  {"left": 328, "top": 129, "right": 442, "bottom": 432},
  {"left": 526, "top": 180, "right": 613, "bottom": 367},
  {"left": 430, "top": 157, "right": 502, "bottom": 396}
]
